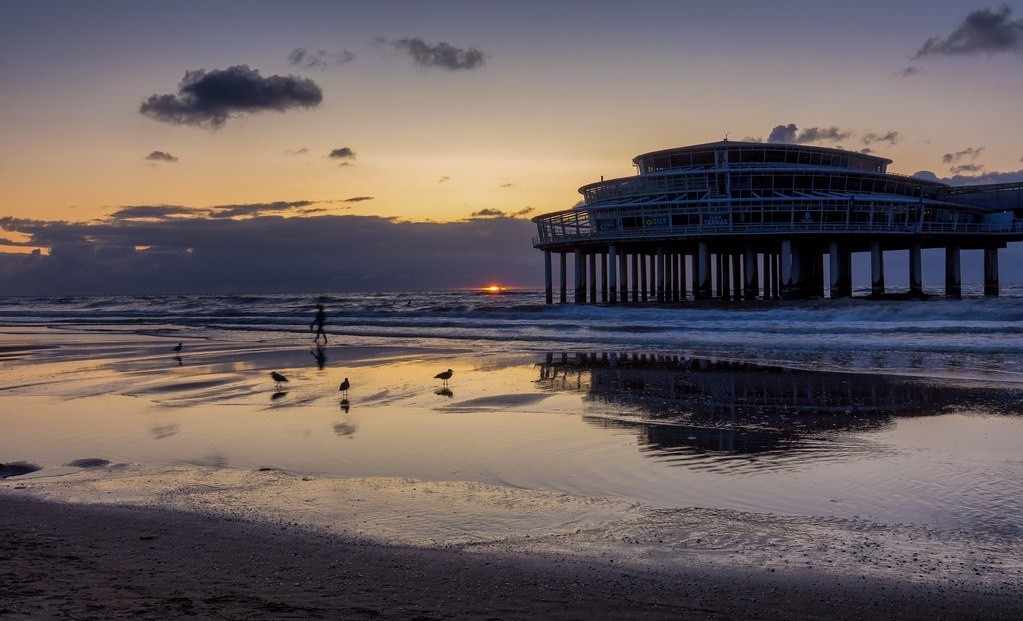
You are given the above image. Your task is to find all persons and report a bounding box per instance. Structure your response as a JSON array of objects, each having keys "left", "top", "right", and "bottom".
[{"left": 310, "top": 304, "right": 328, "bottom": 344}]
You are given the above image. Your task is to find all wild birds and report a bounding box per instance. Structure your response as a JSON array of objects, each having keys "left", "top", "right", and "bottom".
[
  {"left": 171, "top": 342, "right": 184, "bottom": 357},
  {"left": 338, "top": 377, "right": 350, "bottom": 395},
  {"left": 432, "top": 368, "right": 455, "bottom": 385},
  {"left": 268, "top": 370, "right": 290, "bottom": 387}
]
